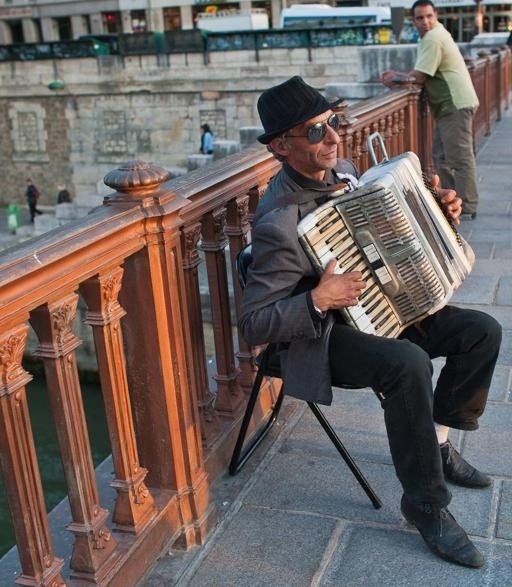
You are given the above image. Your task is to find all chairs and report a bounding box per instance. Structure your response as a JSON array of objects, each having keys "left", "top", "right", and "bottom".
[{"left": 228, "top": 241, "right": 385, "bottom": 510}]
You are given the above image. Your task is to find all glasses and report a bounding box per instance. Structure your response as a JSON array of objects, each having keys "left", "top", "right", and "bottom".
[{"left": 279, "top": 115, "right": 339, "bottom": 144}]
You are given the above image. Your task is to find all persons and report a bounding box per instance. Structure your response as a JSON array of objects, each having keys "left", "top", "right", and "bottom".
[
  {"left": 24, "top": 178, "right": 43, "bottom": 223},
  {"left": 199, "top": 123, "right": 215, "bottom": 155},
  {"left": 378, "top": 0, "right": 480, "bottom": 221},
  {"left": 236, "top": 75, "right": 503, "bottom": 568},
  {"left": 4, "top": 201, "right": 21, "bottom": 236},
  {"left": 55, "top": 182, "right": 71, "bottom": 204}
]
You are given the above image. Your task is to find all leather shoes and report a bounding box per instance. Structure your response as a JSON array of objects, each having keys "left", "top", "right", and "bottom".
[
  {"left": 401, "top": 498, "right": 487, "bottom": 568},
  {"left": 438, "top": 440, "right": 492, "bottom": 486}
]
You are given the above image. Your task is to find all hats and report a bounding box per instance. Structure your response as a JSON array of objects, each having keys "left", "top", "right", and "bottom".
[{"left": 258, "top": 75, "right": 345, "bottom": 147}]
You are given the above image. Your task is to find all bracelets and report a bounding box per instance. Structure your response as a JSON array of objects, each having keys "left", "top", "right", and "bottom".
[{"left": 313, "top": 304, "right": 323, "bottom": 317}]
